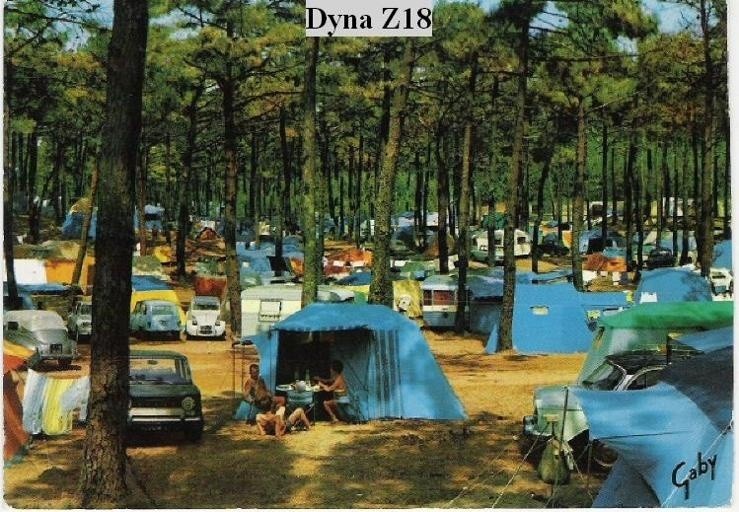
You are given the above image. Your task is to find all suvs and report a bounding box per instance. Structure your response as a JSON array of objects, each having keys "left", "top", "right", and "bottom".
[
  {"left": 526, "top": 351, "right": 696, "bottom": 479},
  {"left": 127, "top": 349, "right": 203, "bottom": 443},
  {"left": 4, "top": 308, "right": 78, "bottom": 367}
]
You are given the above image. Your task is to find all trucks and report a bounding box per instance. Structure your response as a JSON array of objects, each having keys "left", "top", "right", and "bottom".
[{"left": 470, "top": 229, "right": 532, "bottom": 265}]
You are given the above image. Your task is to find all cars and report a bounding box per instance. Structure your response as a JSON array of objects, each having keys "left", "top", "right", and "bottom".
[
  {"left": 131, "top": 299, "right": 183, "bottom": 335},
  {"left": 69, "top": 302, "right": 92, "bottom": 340},
  {"left": 647, "top": 248, "right": 674, "bottom": 268},
  {"left": 185, "top": 296, "right": 226, "bottom": 338}
]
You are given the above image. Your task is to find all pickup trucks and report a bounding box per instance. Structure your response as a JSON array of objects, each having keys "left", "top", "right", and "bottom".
[{"left": 538, "top": 240, "right": 569, "bottom": 255}]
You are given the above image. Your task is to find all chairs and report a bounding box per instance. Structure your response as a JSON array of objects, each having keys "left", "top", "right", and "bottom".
[
  {"left": 333, "top": 384, "right": 360, "bottom": 420},
  {"left": 286, "top": 389, "right": 317, "bottom": 424}
]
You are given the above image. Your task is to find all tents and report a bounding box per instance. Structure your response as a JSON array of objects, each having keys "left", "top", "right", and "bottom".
[{"left": 5, "top": 202, "right": 733, "bottom": 510}]
